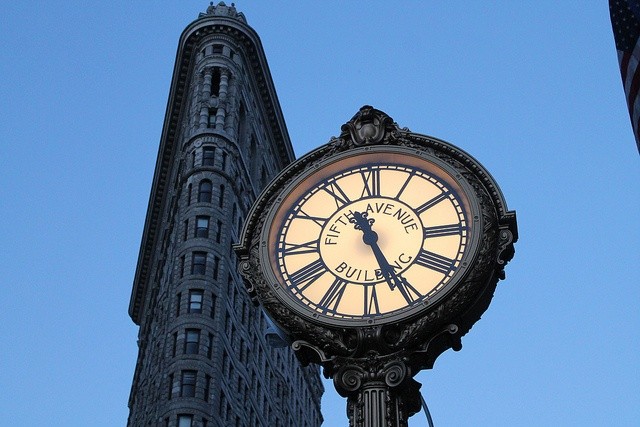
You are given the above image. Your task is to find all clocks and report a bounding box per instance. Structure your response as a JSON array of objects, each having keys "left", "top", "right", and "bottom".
[{"left": 237, "top": 106, "right": 519, "bottom": 371}]
